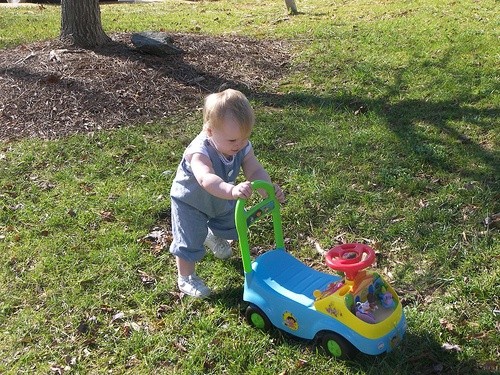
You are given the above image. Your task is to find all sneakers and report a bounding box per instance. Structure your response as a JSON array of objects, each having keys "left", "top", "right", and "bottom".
[
  {"left": 204, "top": 234, "right": 232, "bottom": 259},
  {"left": 178, "top": 278, "right": 209, "bottom": 298}
]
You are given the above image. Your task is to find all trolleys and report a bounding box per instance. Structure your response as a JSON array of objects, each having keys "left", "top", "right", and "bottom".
[{"left": 235, "top": 180, "right": 409, "bottom": 358}]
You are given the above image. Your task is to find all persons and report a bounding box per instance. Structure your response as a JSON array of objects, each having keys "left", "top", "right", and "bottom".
[{"left": 169, "top": 89, "right": 285, "bottom": 298}]
